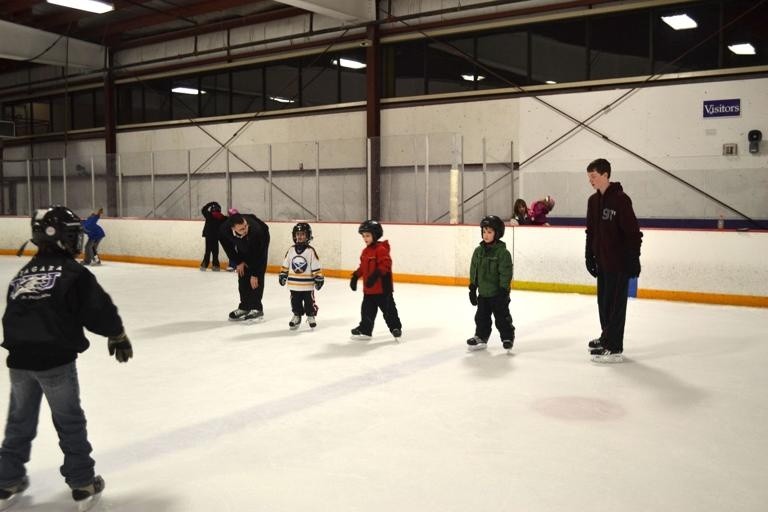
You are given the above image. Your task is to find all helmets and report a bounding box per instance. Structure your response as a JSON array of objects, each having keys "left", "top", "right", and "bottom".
[
  {"left": 479, "top": 215, "right": 505, "bottom": 241},
  {"left": 210, "top": 203, "right": 221, "bottom": 213},
  {"left": 292, "top": 222, "right": 312, "bottom": 244},
  {"left": 31, "top": 205, "right": 83, "bottom": 259},
  {"left": 358, "top": 220, "right": 383, "bottom": 242}
]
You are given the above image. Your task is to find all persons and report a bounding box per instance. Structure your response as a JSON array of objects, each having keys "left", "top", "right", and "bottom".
[
  {"left": 348, "top": 219, "right": 402, "bottom": 339},
  {"left": 278, "top": 221, "right": 326, "bottom": 327},
  {"left": 79, "top": 207, "right": 107, "bottom": 262},
  {"left": 464, "top": 217, "right": 518, "bottom": 350},
  {"left": 581, "top": 160, "right": 643, "bottom": 357},
  {"left": 514, "top": 200, "right": 534, "bottom": 226},
  {"left": 200, "top": 202, "right": 228, "bottom": 268},
  {"left": 221, "top": 215, "right": 269, "bottom": 320},
  {"left": 1, "top": 205, "right": 133, "bottom": 503},
  {"left": 529, "top": 195, "right": 555, "bottom": 226}
]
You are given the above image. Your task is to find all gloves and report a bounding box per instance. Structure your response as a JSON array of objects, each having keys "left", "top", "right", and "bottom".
[
  {"left": 350, "top": 274, "right": 358, "bottom": 291},
  {"left": 469, "top": 290, "right": 478, "bottom": 306},
  {"left": 313, "top": 274, "right": 325, "bottom": 290},
  {"left": 366, "top": 269, "right": 380, "bottom": 288},
  {"left": 279, "top": 272, "right": 287, "bottom": 286},
  {"left": 586, "top": 252, "right": 600, "bottom": 278},
  {"left": 495, "top": 287, "right": 510, "bottom": 312},
  {"left": 625, "top": 253, "right": 640, "bottom": 280}
]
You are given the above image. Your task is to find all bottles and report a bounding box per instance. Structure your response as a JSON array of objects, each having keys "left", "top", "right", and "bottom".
[{"left": 717, "top": 213, "right": 724, "bottom": 231}]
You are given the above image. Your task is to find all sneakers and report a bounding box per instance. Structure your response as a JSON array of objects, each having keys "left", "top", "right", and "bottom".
[
  {"left": 351, "top": 325, "right": 372, "bottom": 336},
  {"left": 226, "top": 266, "right": 234, "bottom": 271},
  {"left": 229, "top": 307, "right": 250, "bottom": 318},
  {"left": 467, "top": 335, "right": 487, "bottom": 345},
  {"left": 502, "top": 339, "right": 513, "bottom": 349},
  {"left": 108, "top": 330, "right": 133, "bottom": 363},
  {"left": 391, "top": 328, "right": 401, "bottom": 337},
  {"left": 307, "top": 316, "right": 317, "bottom": 327},
  {"left": 589, "top": 337, "right": 624, "bottom": 356},
  {"left": 245, "top": 309, "right": 264, "bottom": 320},
  {"left": 289, "top": 314, "right": 301, "bottom": 326},
  {"left": 0, "top": 476, "right": 29, "bottom": 501},
  {"left": 72, "top": 475, "right": 105, "bottom": 501}
]
